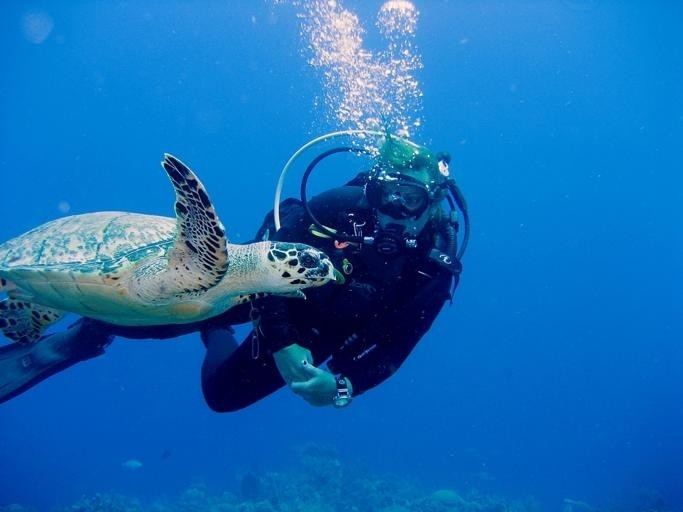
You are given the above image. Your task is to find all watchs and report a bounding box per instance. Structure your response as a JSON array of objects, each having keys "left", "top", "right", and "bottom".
[{"left": 330, "top": 375, "right": 352, "bottom": 409}]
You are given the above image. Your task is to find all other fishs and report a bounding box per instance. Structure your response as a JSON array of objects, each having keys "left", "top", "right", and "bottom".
[
  {"left": 432, "top": 490, "right": 465, "bottom": 506},
  {"left": 121, "top": 459, "right": 143, "bottom": 471}
]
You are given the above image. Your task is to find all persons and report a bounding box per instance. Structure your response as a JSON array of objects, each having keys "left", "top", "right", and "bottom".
[{"left": 30, "top": 129, "right": 470, "bottom": 411}]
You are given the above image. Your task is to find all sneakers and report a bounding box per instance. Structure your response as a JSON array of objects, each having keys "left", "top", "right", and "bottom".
[{"left": 27, "top": 320, "right": 105, "bottom": 370}]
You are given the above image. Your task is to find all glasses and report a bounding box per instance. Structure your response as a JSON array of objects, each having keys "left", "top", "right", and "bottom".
[{"left": 366, "top": 179, "right": 429, "bottom": 216}]
